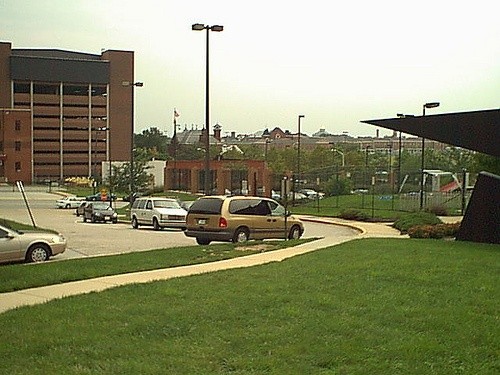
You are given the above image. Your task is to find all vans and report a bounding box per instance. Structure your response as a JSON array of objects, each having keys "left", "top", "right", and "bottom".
[{"left": 183, "top": 195, "right": 305, "bottom": 245}]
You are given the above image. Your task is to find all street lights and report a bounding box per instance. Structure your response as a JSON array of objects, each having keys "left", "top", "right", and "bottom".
[
  {"left": 365, "top": 145, "right": 371, "bottom": 195},
  {"left": 396, "top": 112, "right": 404, "bottom": 197},
  {"left": 297, "top": 115, "right": 305, "bottom": 191},
  {"left": 330, "top": 147, "right": 339, "bottom": 207},
  {"left": 95, "top": 131, "right": 101, "bottom": 194},
  {"left": 420, "top": 102, "right": 440, "bottom": 209},
  {"left": 119, "top": 80, "right": 144, "bottom": 204},
  {"left": 387, "top": 144, "right": 392, "bottom": 194},
  {"left": 190, "top": 22, "right": 225, "bottom": 195}
]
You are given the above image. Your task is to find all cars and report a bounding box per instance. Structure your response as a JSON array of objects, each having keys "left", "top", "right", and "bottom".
[
  {"left": 299, "top": 189, "right": 321, "bottom": 201},
  {"left": 271, "top": 192, "right": 281, "bottom": 199},
  {"left": 287, "top": 191, "right": 307, "bottom": 200},
  {"left": 76, "top": 201, "right": 95, "bottom": 217},
  {"left": 0, "top": 216, "right": 68, "bottom": 265},
  {"left": 85, "top": 192, "right": 118, "bottom": 201},
  {"left": 83, "top": 202, "right": 118, "bottom": 224}
]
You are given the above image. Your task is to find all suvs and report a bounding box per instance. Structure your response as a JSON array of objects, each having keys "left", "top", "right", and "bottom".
[{"left": 130, "top": 197, "right": 189, "bottom": 231}]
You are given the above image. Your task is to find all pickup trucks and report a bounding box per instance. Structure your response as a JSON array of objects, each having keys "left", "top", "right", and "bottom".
[{"left": 56, "top": 196, "right": 87, "bottom": 209}]
